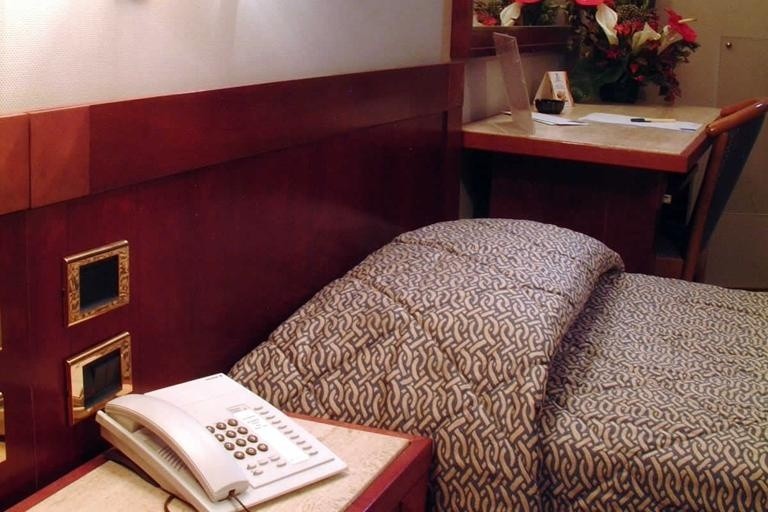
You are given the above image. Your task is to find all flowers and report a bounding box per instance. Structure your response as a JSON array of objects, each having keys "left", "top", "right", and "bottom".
[
  {"left": 476, "top": 0, "right": 576, "bottom": 27},
  {"left": 568, "top": 1, "right": 701, "bottom": 103}
]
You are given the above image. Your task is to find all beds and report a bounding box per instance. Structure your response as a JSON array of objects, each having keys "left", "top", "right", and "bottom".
[{"left": 229, "top": 218, "right": 768, "bottom": 512}]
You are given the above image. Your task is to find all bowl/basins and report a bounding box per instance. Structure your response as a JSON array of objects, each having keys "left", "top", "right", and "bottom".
[{"left": 535, "top": 99, "right": 565, "bottom": 115}]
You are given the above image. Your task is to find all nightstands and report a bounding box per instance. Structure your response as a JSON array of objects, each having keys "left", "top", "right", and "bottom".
[{"left": 0, "top": 410, "right": 434, "bottom": 512}]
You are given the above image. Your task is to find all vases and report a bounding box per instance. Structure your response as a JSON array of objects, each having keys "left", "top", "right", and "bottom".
[{"left": 601, "top": 75, "right": 639, "bottom": 104}]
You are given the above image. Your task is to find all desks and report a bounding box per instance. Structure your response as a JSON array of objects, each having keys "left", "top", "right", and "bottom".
[{"left": 461, "top": 103, "right": 724, "bottom": 279}]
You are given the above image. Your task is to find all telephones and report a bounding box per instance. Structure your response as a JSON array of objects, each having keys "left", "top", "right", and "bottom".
[{"left": 93, "top": 370, "right": 348, "bottom": 512}]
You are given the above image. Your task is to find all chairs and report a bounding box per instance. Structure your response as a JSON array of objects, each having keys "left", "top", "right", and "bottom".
[{"left": 641, "top": 96, "right": 768, "bottom": 284}]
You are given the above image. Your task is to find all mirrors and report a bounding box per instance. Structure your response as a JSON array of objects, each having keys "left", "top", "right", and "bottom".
[{"left": 450, "top": 0, "right": 582, "bottom": 61}]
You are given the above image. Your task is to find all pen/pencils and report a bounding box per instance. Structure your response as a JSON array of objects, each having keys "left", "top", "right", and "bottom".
[{"left": 630, "top": 115, "right": 677, "bottom": 123}]
